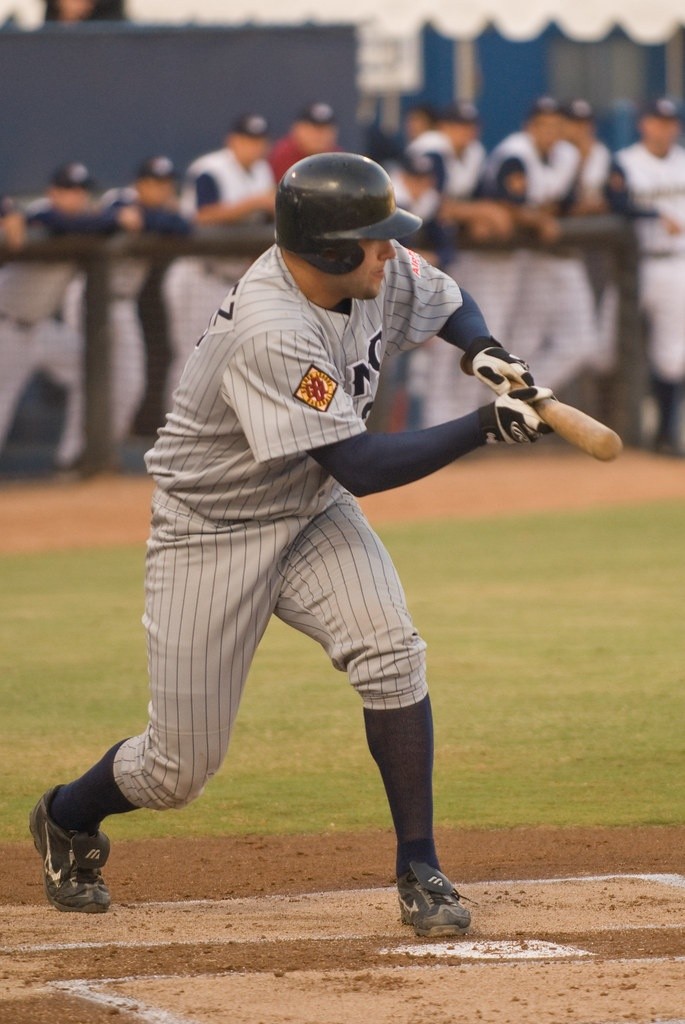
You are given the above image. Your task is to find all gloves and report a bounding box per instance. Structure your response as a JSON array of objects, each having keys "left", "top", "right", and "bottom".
[
  {"left": 479, "top": 386, "right": 555, "bottom": 445},
  {"left": 470, "top": 338, "right": 536, "bottom": 396}
]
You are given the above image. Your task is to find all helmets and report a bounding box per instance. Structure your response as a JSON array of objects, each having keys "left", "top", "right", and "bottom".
[{"left": 275, "top": 152, "right": 423, "bottom": 274}]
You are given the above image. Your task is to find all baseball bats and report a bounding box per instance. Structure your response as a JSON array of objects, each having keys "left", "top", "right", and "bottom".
[{"left": 510, "top": 381, "right": 623, "bottom": 461}]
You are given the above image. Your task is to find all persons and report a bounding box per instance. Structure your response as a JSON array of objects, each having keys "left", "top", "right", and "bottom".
[
  {"left": 0, "top": 94, "right": 685, "bottom": 487},
  {"left": 29, "top": 152, "right": 555, "bottom": 941}
]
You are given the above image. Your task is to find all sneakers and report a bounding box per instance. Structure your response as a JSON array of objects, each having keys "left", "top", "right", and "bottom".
[
  {"left": 29, "top": 784, "right": 111, "bottom": 913},
  {"left": 396, "top": 861, "right": 471, "bottom": 937}
]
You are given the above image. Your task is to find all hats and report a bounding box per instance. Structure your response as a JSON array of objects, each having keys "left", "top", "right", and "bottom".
[
  {"left": 402, "top": 152, "right": 435, "bottom": 176},
  {"left": 233, "top": 112, "right": 277, "bottom": 136},
  {"left": 644, "top": 98, "right": 681, "bottom": 121},
  {"left": 445, "top": 99, "right": 480, "bottom": 125},
  {"left": 300, "top": 101, "right": 339, "bottom": 125},
  {"left": 51, "top": 164, "right": 95, "bottom": 189},
  {"left": 138, "top": 157, "right": 180, "bottom": 178},
  {"left": 533, "top": 97, "right": 564, "bottom": 112},
  {"left": 564, "top": 98, "right": 593, "bottom": 122}
]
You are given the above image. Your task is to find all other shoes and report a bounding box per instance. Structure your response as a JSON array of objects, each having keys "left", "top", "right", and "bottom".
[{"left": 648, "top": 372, "right": 684, "bottom": 457}]
